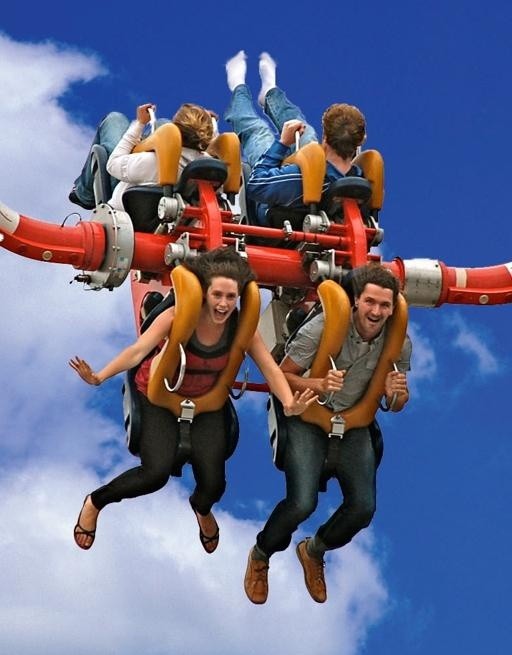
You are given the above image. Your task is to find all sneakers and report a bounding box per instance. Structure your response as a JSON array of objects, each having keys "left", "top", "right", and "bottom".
[
  {"left": 296, "top": 538, "right": 326, "bottom": 603},
  {"left": 69, "top": 189, "right": 96, "bottom": 210},
  {"left": 244, "top": 545, "right": 269, "bottom": 604}
]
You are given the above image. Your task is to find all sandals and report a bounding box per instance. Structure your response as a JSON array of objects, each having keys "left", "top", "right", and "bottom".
[
  {"left": 74, "top": 494, "right": 101, "bottom": 551},
  {"left": 189, "top": 493, "right": 219, "bottom": 554}
]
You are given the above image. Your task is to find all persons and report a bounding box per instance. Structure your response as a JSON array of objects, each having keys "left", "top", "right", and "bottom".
[
  {"left": 224, "top": 48, "right": 370, "bottom": 244},
  {"left": 243, "top": 263, "right": 416, "bottom": 607},
  {"left": 67, "top": 100, "right": 247, "bottom": 240},
  {"left": 65, "top": 245, "right": 322, "bottom": 555}
]
